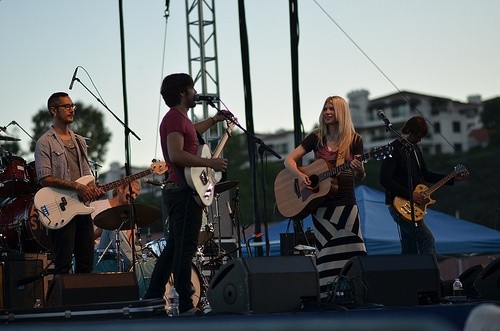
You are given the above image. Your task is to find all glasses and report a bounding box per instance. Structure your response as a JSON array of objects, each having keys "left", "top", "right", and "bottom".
[{"left": 54, "top": 104, "right": 76, "bottom": 110}]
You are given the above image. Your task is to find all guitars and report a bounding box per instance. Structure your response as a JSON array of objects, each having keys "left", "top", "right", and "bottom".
[
  {"left": 184, "top": 117, "right": 238, "bottom": 207},
  {"left": 393, "top": 166, "right": 472, "bottom": 223},
  {"left": 274, "top": 143, "right": 395, "bottom": 221},
  {"left": 33, "top": 158, "right": 169, "bottom": 230}
]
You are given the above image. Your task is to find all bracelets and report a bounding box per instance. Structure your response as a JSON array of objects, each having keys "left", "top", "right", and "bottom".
[{"left": 212, "top": 118, "right": 215, "bottom": 126}]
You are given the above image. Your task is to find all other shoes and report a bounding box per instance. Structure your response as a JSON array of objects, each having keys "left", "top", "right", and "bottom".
[{"left": 179, "top": 307, "right": 200, "bottom": 316}]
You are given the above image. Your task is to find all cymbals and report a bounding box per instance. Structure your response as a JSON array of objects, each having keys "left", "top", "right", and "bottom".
[
  {"left": 93, "top": 203, "right": 162, "bottom": 230},
  {"left": 214, "top": 181, "right": 240, "bottom": 194},
  {"left": 0, "top": 136, "right": 21, "bottom": 141}
]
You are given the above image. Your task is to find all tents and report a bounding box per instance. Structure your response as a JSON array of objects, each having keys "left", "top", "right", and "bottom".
[{"left": 242, "top": 184, "right": 500, "bottom": 278}]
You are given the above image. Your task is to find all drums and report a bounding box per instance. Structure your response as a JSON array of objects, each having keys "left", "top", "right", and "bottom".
[
  {"left": 0, "top": 156, "right": 32, "bottom": 191},
  {"left": 0, "top": 194, "right": 49, "bottom": 252},
  {"left": 163, "top": 208, "right": 215, "bottom": 247},
  {"left": 128, "top": 256, "right": 202, "bottom": 309},
  {"left": 70, "top": 249, "right": 125, "bottom": 274},
  {"left": 27, "top": 160, "right": 43, "bottom": 193},
  {"left": 141, "top": 236, "right": 169, "bottom": 258}
]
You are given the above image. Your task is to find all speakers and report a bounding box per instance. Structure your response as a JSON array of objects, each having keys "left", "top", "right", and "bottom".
[
  {"left": 48, "top": 273, "right": 139, "bottom": 306},
  {"left": 0, "top": 259, "right": 46, "bottom": 314},
  {"left": 207, "top": 256, "right": 320, "bottom": 315},
  {"left": 331, "top": 253, "right": 500, "bottom": 311}
]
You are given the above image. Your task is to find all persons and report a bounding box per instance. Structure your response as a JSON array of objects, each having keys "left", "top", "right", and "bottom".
[
  {"left": 90, "top": 180, "right": 140, "bottom": 271},
  {"left": 34, "top": 92, "right": 105, "bottom": 274},
  {"left": 283, "top": 96, "right": 366, "bottom": 312},
  {"left": 135, "top": 74, "right": 232, "bottom": 317},
  {"left": 380, "top": 117, "right": 469, "bottom": 256}
]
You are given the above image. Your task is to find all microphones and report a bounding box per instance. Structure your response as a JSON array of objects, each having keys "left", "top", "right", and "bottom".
[
  {"left": 377, "top": 110, "right": 392, "bottom": 127},
  {"left": 194, "top": 94, "right": 220, "bottom": 101},
  {"left": 142, "top": 178, "right": 162, "bottom": 186},
  {"left": 69, "top": 67, "right": 78, "bottom": 89},
  {"left": 226, "top": 201, "right": 236, "bottom": 227}
]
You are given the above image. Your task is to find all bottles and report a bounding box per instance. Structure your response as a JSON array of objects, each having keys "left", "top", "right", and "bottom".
[
  {"left": 34, "top": 299, "right": 43, "bottom": 308},
  {"left": 168, "top": 288, "right": 179, "bottom": 317},
  {"left": 453, "top": 279, "right": 463, "bottom": 296}
]
